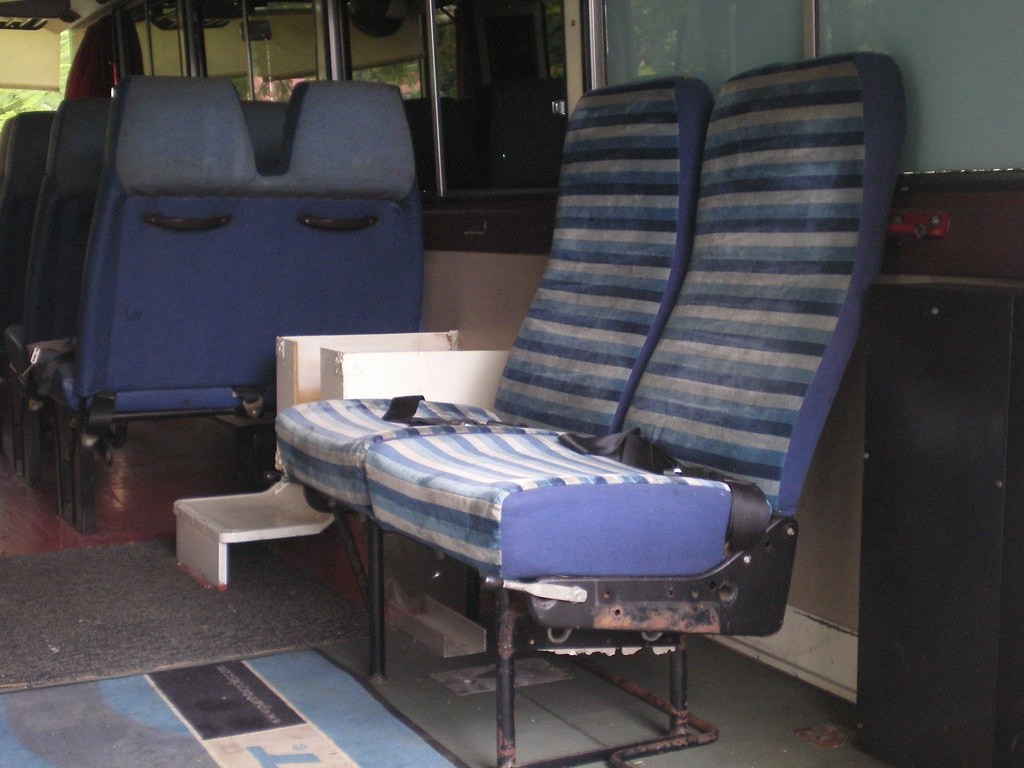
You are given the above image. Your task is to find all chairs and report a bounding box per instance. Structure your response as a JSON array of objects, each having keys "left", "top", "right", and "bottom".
[
  {"left": 275, "top": 74, "right": 716, "bottom": 694},
  {"left": 363, "top": 49, "right": 908, "bottom": 768}
]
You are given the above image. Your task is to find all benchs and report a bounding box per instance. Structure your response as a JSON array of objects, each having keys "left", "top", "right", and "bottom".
[{"left": 0, "top": 73, "right": 424, "bottom": 537}]
